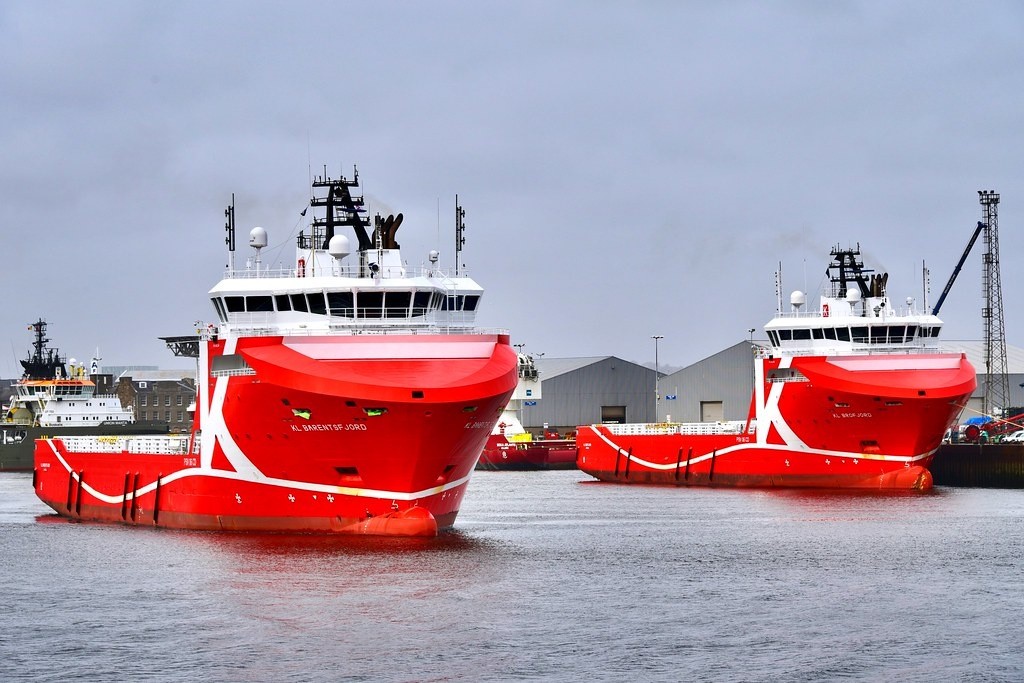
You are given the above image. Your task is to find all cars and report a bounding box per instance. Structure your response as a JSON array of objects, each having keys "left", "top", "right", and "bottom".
[{"left": 1000, "top": 430, "right": 1024, "bottom": 444}]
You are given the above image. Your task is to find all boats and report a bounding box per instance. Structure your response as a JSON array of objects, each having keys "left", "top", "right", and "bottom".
[
  {"left": 0, "top": 318, "right": 170, "bottom": 473},
  {"left": 474, "top": 355, "right": 580, "bottom": 472},
  {"left": 33, "top": 128, "right": 519, "bottom": 537},
  {"left": 575, "top": 243, "right": 977, "bottom": 492}
]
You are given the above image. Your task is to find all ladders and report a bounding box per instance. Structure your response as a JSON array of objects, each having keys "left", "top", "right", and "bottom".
[{"left": 986, "top": 413, "right": 1024, "bottom": 435}]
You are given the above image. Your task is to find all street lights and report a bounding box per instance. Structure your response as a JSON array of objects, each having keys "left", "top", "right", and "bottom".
[
  {"left": 513, "top": 344, "right": 525, "bottom": 354},
  {"left": 650, "top": 335, "right": 664, "bottom": 422}
]
